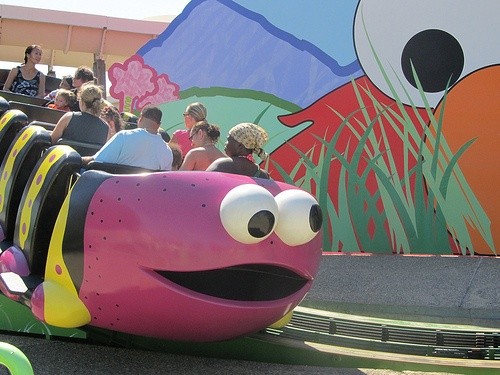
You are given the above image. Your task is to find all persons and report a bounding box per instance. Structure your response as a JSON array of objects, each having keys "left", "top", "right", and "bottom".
[
  {"left": 43, "top": 67, "right": 269, "bottom": 180},
  {"left": 3, "top": 44, "right": 45, "bottom": 98}
]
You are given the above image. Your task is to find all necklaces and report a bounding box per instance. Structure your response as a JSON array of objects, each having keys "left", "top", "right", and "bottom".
[{"left": 234, "top": 155, "right": 248, "bottom": 160}]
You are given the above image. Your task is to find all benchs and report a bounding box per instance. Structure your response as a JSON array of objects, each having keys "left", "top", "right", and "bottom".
[{"left": 0, "top": 68, "right": 172, "bottom": 175}]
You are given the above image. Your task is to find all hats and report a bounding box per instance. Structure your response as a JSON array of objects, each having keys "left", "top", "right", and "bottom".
[{"left": 228, "top": 122, "right": 269, "bottom": 149}]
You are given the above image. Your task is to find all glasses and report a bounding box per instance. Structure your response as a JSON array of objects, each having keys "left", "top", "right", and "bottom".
[
  {"left": 183, "top": 112, "right": 190, "bottom": 116},
  {"left": 189, "top": 132, "right": 196, "bottom": 142}
]
